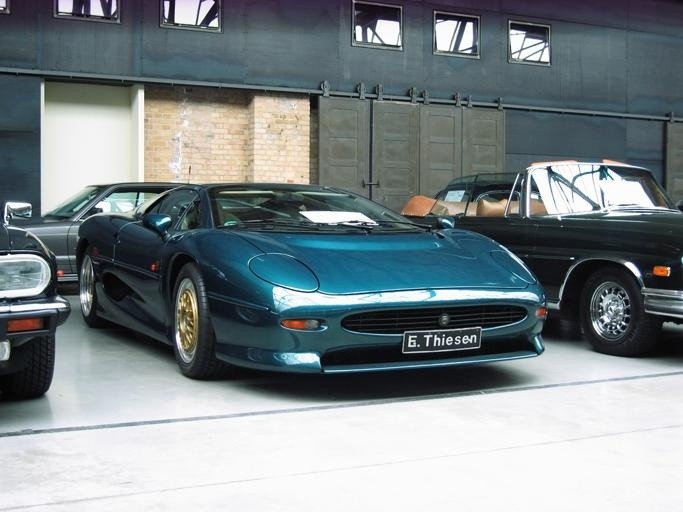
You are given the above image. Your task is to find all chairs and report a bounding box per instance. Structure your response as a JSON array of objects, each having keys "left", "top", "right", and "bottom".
[
  {"left": 478, "top": 197, "right": 511, "bottom": 218},
  {"left": 181, "top": 200, "right": 244, "bottom": 229}
]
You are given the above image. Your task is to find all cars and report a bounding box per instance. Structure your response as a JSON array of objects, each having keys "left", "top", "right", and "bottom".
[
  {"left": 10, "top": 180, "right": 185, "bottom": 296},
  {"left": 1, "top": 197, "right": 73, "bottom": 403},
  {"left": 403, "top": 157, "right": 683, "bottom": 357}
]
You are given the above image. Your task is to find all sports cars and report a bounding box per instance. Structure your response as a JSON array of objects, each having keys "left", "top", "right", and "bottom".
[{"left": 74, "top": 181, "right": 551, "bottom": 385}]
